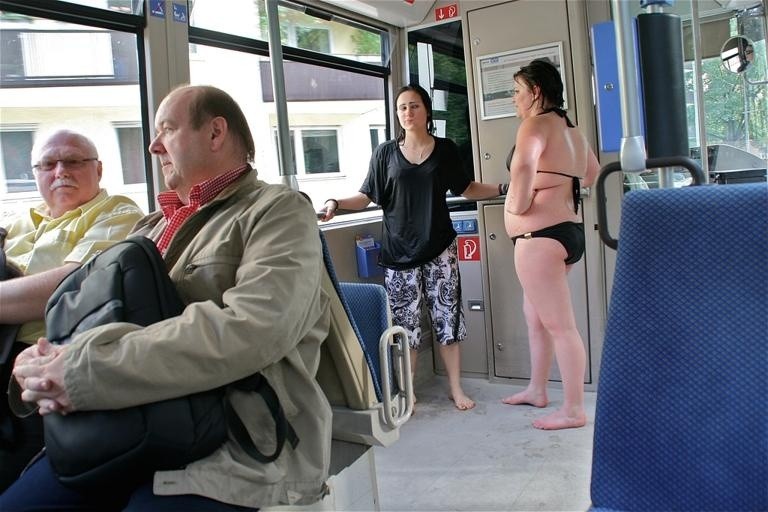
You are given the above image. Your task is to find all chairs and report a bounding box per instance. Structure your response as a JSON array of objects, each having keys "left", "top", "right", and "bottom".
[
  {"left": 585, "top": 179, "right": 768, "bottom": 511},
  {"left": 317, "top": 224, "right": 415, "bottom": 450}
]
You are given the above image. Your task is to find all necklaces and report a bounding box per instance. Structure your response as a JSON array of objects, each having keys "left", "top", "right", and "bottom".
[{"left": 407, "top": 145, "right": 428, "bottom": 160}]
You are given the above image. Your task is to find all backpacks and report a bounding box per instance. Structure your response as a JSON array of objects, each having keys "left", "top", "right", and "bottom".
[{"left": 36, "top": 233, "right": 301, "bottom": 492}]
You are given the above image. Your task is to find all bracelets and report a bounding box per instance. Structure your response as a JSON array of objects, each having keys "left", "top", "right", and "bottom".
[
  {"left": 499, "top": 182, "right": 510, "bottom": 197},
  {"left": 324, "top": 198, "right": 339, "bottom": 213}
]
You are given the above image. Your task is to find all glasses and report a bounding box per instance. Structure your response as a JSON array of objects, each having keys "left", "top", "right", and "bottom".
[{"left": 31, "top": 156, "right": 98, "bottom": 172}]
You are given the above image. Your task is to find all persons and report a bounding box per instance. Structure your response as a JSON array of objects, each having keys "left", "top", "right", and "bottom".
[
  {"left": 317, "top": 84, "right": 513, "bottom": 412},
  {"left": 736, "top": 43, "right": 754, "bottom": 73},
  {"left": 0, "top": 83, "right": 333, "bottom": 510},
  {"left": 501, "top": 55, "right": 601, "bottom": 431},
  {"left": 0, "top": 124, "right": 146, "bottom": 500}
]
